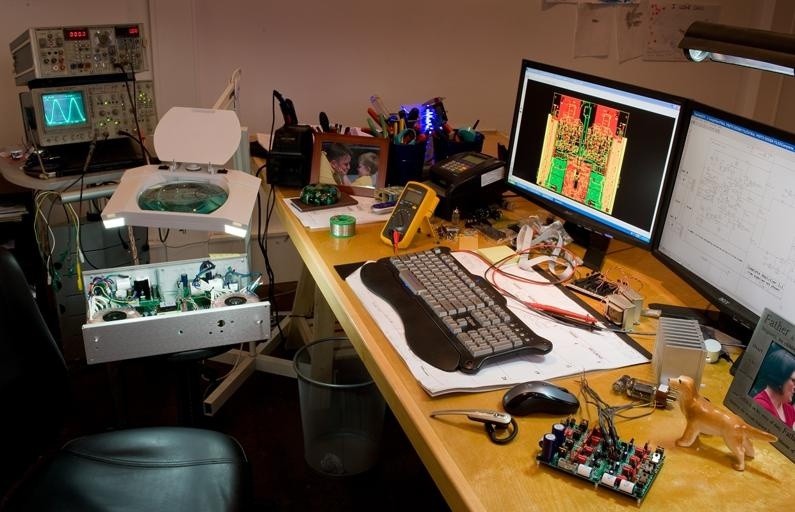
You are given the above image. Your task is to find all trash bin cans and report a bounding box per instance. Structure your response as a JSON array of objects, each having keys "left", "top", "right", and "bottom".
[{"left": 293, "top": 336, "right": 386, "bottom": 477}]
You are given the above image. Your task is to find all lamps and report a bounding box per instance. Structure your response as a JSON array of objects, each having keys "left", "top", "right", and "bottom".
[{"left": 675, "top": 19, "right": 795, "bottom": 81}]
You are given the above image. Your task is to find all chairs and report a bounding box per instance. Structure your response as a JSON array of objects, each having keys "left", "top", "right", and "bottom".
[{"left": 1, "top": 250, "right": 251, "bottom": 512}]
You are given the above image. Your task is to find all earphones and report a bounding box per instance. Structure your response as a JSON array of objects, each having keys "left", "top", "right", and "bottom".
[{"left": 430, "top": 411, "right": 512, "bottom": 430}]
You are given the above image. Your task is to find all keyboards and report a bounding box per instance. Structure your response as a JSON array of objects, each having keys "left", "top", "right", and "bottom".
[{"left": 360, "top": 245, "right": 552, "bottom": 373}]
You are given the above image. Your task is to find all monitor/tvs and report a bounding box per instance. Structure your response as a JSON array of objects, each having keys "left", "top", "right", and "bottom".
[
  {"left": 649, "top": 99, "right": 794, "bottom": 348},
  {"left": 503, "top": 59, "right": 688, "bottom": 271}
]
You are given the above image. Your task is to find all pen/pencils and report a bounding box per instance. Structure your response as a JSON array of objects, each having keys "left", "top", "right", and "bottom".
[{"left": 312, "top": 96, "right": 426, "bottom": 144}]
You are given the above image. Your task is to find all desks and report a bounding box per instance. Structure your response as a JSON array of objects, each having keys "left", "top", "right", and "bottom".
[{"left": 247, "top": 129, "right": 794, "bottom": 512}]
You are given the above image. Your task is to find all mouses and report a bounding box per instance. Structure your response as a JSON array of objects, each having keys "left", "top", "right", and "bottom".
[{"left": 503, "top": 380, "right": 580, "bottom": 417}]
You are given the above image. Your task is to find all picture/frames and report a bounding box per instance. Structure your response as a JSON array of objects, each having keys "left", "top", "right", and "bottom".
[
  {"left": 720, "top": 306, "right": 794, "bottom": 464},
  {"left": 306, "top": 132, "right": 391, "bottom": 199}
]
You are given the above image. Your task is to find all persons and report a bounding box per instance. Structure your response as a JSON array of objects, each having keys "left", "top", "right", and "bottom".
[
  {"left": 319, "top": 143, "right": 353, "bottom": 185},
  {"left": 752, "top": 347, "right": 795, "bottom": 431},
  {"left": 336, "top": 151, "right": 378, "bottom": 188}
]
